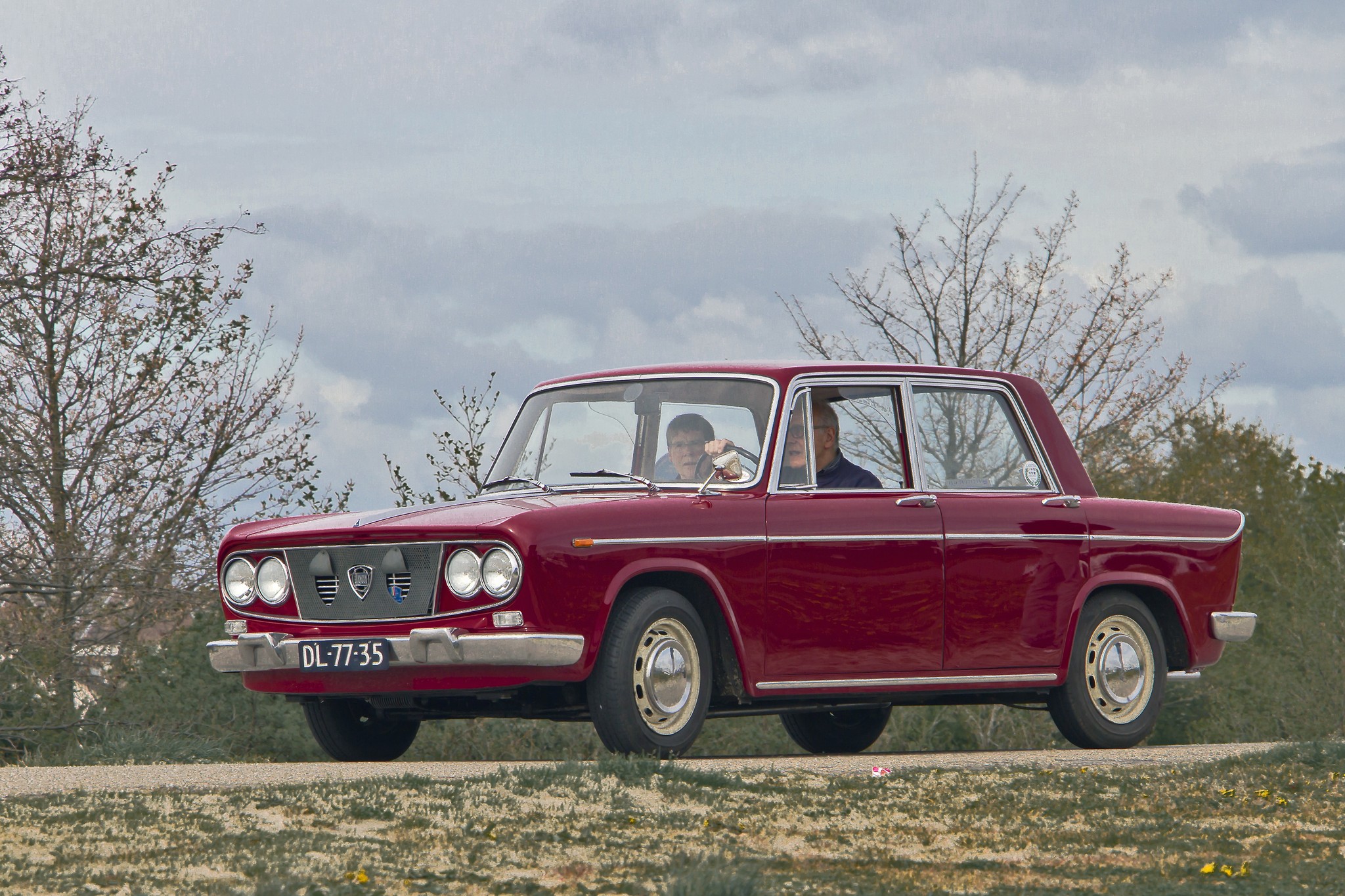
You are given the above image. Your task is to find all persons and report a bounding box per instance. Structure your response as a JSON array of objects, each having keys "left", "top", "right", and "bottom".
[
  {"left": 705, "top": 400, "right": 884, "bottom": 489},
  {"left": 666, "top": 414, "right": 716, "bottom": 481}
]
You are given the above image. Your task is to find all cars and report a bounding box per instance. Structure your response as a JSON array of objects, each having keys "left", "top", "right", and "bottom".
[{"left": 203, "top": 360, "right": 1262, "bottom": 761}]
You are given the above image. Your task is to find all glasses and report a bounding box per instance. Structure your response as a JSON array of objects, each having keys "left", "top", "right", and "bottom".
[
  {"left": 670, "top": 441, "right": 710, "bottom": 454},
  {"left": 788, "top": 425, "right": 832, "bottom": 439}
]
